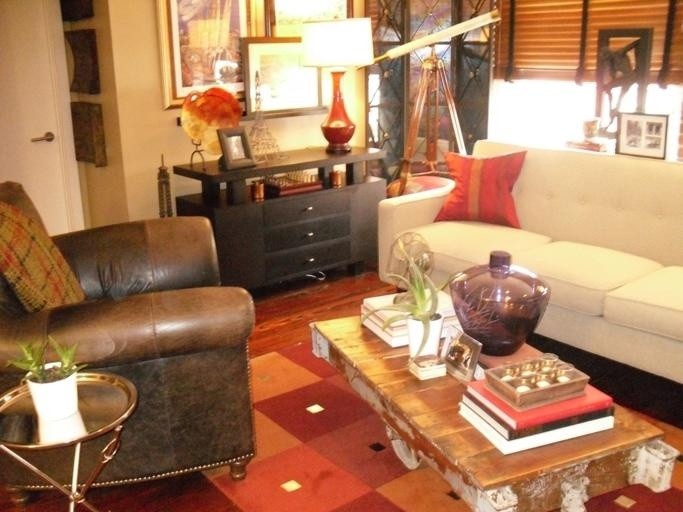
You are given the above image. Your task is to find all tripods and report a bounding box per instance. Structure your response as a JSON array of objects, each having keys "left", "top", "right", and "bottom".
[{"left": 398, "top": 58, "right": 467, "bottom": 196}]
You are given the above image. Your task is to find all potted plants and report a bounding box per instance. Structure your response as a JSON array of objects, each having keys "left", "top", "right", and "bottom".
[
  {"left": 361, "top": 240, "right": 465, "bottom": 358},
  {"left": 5, "top": 335, "right": 87, "bottom": 444}
]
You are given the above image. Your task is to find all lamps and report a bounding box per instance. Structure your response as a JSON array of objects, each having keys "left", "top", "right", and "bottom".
[{"left": 304, "top": 18, "right": 373, "bottom": 152}]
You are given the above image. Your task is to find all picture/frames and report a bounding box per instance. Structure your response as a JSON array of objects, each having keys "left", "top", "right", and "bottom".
[
  {"left": 155, "top": 0, "right": 276, "bottom": 111},
  {"left": 615, "top": 112, "right": 668, "bottom": 159},
  {"left": 241, "top": 37, "right": 324, "bottom": 115},
  {"left": 217, "top": 128, "right": 255, "bottom": 170}
]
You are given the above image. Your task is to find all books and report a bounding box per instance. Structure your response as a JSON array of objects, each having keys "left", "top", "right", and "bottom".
[
  {"left": 458, "top": 378, "right": 615, "bottom": 457},
  {"left": 567, "top": 136, "right": 612, "bottom": 154},
  {"left": 359, "top": 286, "right": 465, "bottom": 349}
]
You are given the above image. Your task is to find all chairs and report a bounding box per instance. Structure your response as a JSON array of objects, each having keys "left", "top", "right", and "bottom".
[{"left": 0, "top": 180, "right": 255, "bottom": 490}]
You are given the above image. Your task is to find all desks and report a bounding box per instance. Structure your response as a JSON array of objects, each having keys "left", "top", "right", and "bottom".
[{"left": 173, "top": 146, "right": 386, "bottom": 299}]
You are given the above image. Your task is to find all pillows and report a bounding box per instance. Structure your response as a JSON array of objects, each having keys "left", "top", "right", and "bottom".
[
  {"left": 1, "top": 202, "right": 85, "bottom": 312},
  {"left": 434, "top": 148, "right": 527, "bottom": 230}
]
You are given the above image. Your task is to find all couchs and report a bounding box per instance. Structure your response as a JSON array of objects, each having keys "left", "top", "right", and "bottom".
[{"left": 378, "top": 139, "right": 683, "bottom": 383}]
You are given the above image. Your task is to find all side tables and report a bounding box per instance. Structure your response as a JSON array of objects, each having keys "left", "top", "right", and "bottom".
[{"left": 1, "top": 372, "right": 138, "bottom": 512}]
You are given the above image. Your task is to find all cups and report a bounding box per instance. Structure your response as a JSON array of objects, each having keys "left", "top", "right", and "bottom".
[
  {"left": 499, "top": 354, "right": 574, "bottom": 394},
  {"left": 264, "top": 169, "right": 314, "bottom": 187},
  {"left": 633, "top": 438, "right": 680, "bottom": 494},
  {"left": 582, "top": 117, "right": 600, "bottom": 140},
  {"left": 327, "top": 171, "right": 342, "bottom": 187},
  {"left": 249, "top": 182, "right": 264, "bottom": 200}
]
